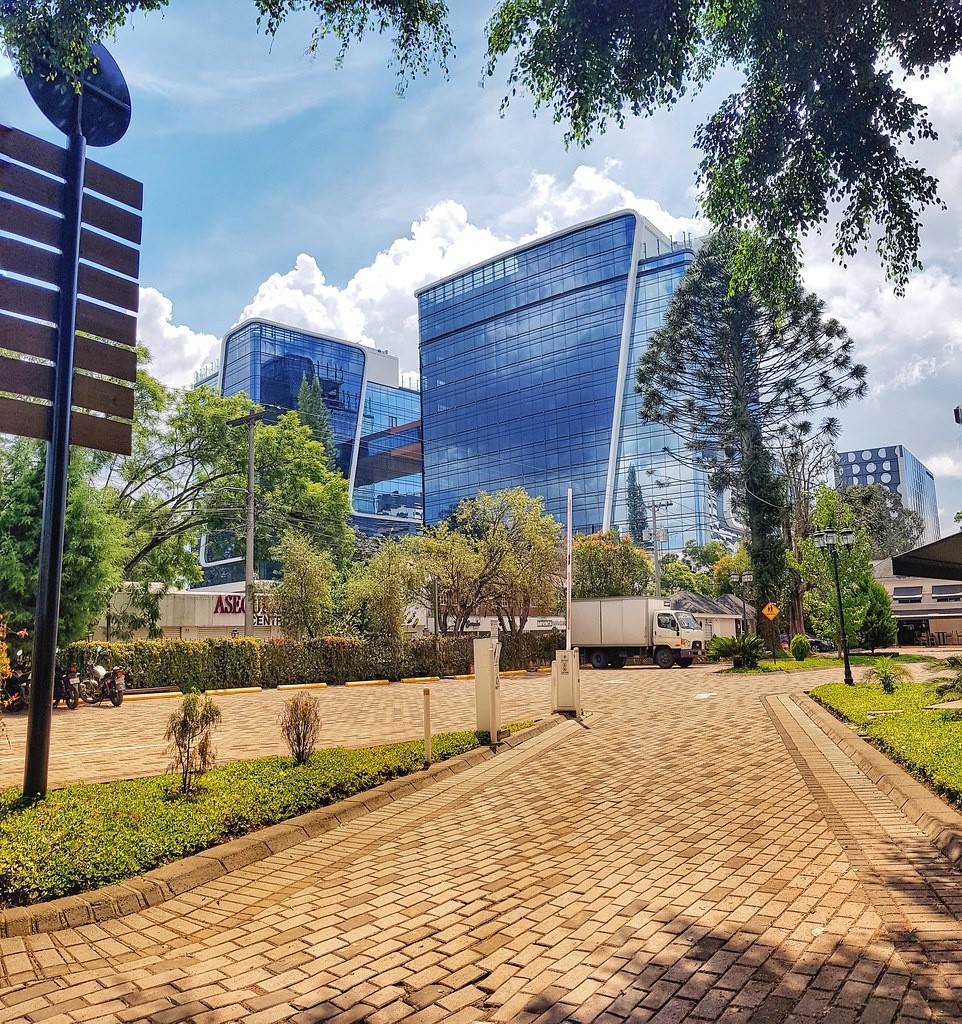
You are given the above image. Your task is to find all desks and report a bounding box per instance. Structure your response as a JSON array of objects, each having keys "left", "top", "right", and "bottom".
[{"left": 935, "top": 632, "right": 947, "bottom": 645}]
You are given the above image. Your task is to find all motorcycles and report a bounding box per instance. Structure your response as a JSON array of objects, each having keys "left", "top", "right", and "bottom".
[{"left": 1, "top": 646, "right": 126, "bottom": 712}]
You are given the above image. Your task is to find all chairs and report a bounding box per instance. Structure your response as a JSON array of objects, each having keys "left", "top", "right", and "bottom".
[
  {"left": 927, "top": 631, "right": 936, "bottom": 646},
  {"left": 945, "top": 630, "right": 953, "bottom": 645},
  {"left": 956, "top": 630, "right": 962, "bottom": 645}
]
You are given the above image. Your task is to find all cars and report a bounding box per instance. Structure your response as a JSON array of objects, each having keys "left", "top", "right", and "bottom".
[{"left": 781, "top": 632, "right": 834, "bottom": 653}]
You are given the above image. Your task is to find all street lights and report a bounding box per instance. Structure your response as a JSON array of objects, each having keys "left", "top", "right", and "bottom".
[
  {"left": 812, "top": 520, "right": 855, "bottom": 688},
  {"left": 731, "top": 568, "right": 753, "bottom": 639}
]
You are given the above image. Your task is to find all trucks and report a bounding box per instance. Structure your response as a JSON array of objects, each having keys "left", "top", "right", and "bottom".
[{"left": 571, "top": 596, "right": 706, "bottom": 669}]
